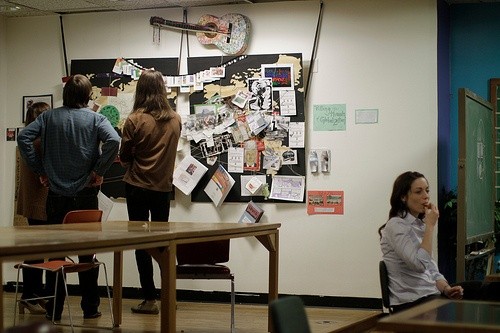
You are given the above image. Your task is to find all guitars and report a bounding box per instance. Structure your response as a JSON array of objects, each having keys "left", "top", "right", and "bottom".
[{"left": 149, "top": 14, "right": 251, "bottom": 56}]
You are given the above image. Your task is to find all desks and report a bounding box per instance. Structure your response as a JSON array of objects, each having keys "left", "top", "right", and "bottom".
[
  {"left": 0, "top": 220, "right": 282, "bottom": 333},
  {"left": 328, "top": 296, "right": 500, "bottom": 333}
]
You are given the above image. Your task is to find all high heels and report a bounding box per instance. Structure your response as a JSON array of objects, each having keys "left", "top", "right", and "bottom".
[{"left": 18, "top": 299, "right": 47, "bottom": 314}]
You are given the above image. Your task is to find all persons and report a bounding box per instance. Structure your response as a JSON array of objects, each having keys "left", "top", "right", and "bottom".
[
  {"left": 14, "top": 76, "right": 121, "bottom": 318},
  {"left": 378, "top": 170, "right": 463, "bottom": 313},
  {"left": 118, "top": 70, "right": 177, "bottom": 314}
]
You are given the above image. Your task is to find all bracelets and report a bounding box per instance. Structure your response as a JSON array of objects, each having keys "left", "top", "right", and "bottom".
[{"left": 442, "top": 284, "right": 450, "bottom": 294}]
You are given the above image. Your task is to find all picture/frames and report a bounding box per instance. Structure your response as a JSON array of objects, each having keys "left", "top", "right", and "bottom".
[{"left": 22, "top": 94, "right": 53, "bottom": 123}]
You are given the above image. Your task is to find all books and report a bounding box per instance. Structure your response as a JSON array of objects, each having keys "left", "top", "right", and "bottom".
[{"left": 172, "top": 155, "right": 264, "bottom": 224}]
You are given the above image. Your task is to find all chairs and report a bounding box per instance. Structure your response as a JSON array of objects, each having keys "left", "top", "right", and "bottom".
[
  {"left": 266, "top": 296, "right": 312, "bottom": 333},
  {"left": 379, "top": 261, "right": 391, "bottom": 315},
  {"left": 13, "top": 209, "right": 115, "bottom": 333},
  {"left": 176, "top": 238, "right": 235, "bottom": 333}
]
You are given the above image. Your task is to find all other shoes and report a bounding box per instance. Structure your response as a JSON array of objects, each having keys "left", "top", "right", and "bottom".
[
  {"left": 47, "top": 315, "right": 60, "bottom": 321},
  {"left": 131, "top": 299, "right": 160, "bottom": 314},
  {"left": 84, "top": 311, "right": 101, "bottom": 319}
]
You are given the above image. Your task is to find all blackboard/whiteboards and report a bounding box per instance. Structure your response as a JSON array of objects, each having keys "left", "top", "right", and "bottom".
[
  {"left": 455, "top": 86, "right": 496, "bottom": 246},
  {"left": 67, "top": 51, "right": 307, "bottom": 204}
]
